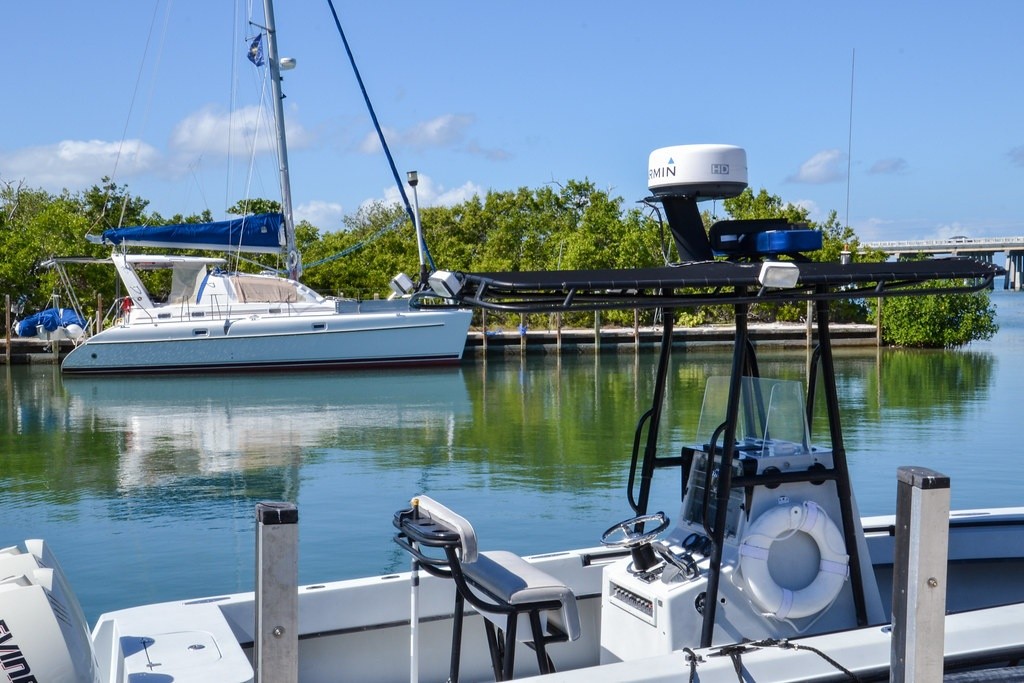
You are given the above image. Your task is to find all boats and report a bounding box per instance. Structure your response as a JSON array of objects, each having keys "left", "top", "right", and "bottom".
[{"left": 15, "top": 306, "right": 89, "bottom": 341}]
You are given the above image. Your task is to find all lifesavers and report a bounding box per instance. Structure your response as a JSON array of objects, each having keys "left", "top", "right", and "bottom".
[
  {"left": 739, "top": 503, "right": 848, "bottom": 619},
  {"left": 123, "top": 299, "right": 131, "bottom": 313}
]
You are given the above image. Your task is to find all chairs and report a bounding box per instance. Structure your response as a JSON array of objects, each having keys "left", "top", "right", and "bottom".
[{"left": 411, "top": 494, "right": 580, "bottom": 683}]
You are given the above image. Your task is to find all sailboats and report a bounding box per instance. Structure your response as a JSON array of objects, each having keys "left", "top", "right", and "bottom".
[{"left": 38, "top": 0, "right": 474, "bottom": 375}]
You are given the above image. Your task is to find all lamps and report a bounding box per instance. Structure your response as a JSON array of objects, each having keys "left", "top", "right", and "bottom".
[
  {"left": 758, "top": 262, "right": 799, "bottom": 298},
  {"left": 407, "top": 171, "right": 418, "bottom": 186},
  {"left": 429, "top": 270, "right": 467, "bottom": 298},
  {"left": 389, "top": 273, "right": 418, "bottom": 296}
]
